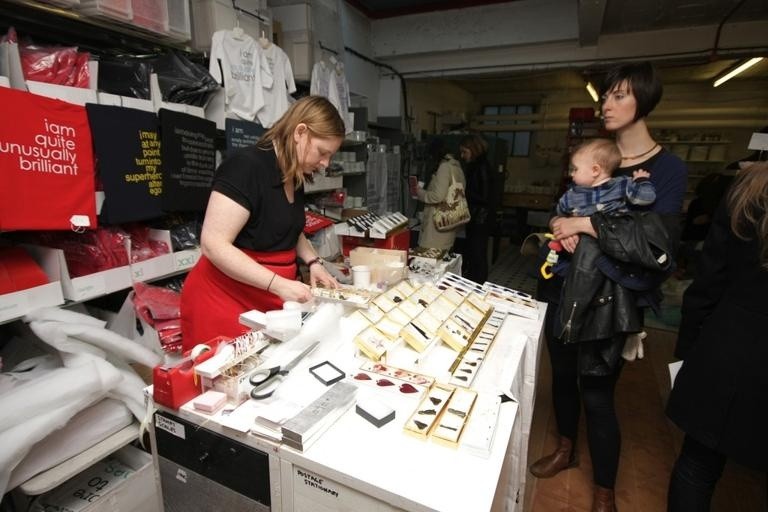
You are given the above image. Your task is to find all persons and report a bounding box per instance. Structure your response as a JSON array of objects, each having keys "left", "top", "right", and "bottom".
[
  {"left": 409, "top": 137, "right": 494, "bottom": 250},
  {"left": 666, "top": 127, "right": 768, "bottom": 512},
  {"left": 551, "top": 137, "right": 656, "bottom": 216},
  {"left": 180, "top": 96, "right": 345, "bottom": 357},
  {"left": 529, "top": 64, "right": 688, "bottom": 512}
]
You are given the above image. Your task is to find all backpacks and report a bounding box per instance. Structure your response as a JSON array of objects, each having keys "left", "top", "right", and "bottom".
[{"left": 489, "top": 167, "right": 506, "bottom": 208}]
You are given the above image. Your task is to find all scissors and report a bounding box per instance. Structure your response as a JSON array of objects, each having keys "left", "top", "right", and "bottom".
[{"left": 248, "top": 339, "right": 320, "bottom": 399}]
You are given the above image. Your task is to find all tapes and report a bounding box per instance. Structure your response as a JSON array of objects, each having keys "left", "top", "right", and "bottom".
[{"left": 190, "top": 344, "right": 212, "bottom": 361}]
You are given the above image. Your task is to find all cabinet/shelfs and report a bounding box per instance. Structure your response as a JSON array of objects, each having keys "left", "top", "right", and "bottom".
[
  {"left": 2, "top": 0, "right": 229, "bottom": 497},
  {"left": 655, "top": 136, "right": 733, "bottom": 214}
]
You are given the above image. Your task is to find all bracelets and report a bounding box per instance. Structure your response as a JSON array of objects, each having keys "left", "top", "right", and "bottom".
[
  {"left": 266, "top": 272, "right": 277, "bottom": 292},
  {"left": 308, "top": 257, "right": 324, "bottom": 271}
]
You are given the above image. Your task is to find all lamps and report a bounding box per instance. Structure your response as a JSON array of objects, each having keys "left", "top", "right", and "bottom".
[
  {"left": 710, "top": 57, "right": 765, "bottom": 89},
  {"left": 585, "top": 80, "right": 601, "bottom": 104}
]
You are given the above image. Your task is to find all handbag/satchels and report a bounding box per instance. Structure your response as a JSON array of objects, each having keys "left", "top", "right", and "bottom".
[{"left": 433, "top": 182, "right": 472, "bottom": 232}]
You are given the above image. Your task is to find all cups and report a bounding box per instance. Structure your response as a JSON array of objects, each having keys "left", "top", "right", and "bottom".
[
  {"left": 347, "top": 196, "right": 365, "bottom": 208},
  {"left": 341, "top": 151, "right": 365, "bottom": 172},
  {"left": 351, "top": 130, "right": 370, "bottom": 141},
  {"left": 351, "top": 264, "right": 371, "bottom": 288}
]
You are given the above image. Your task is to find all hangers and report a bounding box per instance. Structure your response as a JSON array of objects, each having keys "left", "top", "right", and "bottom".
[
  {"left": 229, "top": 19, "right": 274, "bottom": 50},
  {"left": 317, "top": 47, "right": 344, "bottom": 76}
]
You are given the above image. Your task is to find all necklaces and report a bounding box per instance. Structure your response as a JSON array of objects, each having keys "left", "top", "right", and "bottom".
[{"left": 621, "top": 143, "right": 658, "bottom": 160}]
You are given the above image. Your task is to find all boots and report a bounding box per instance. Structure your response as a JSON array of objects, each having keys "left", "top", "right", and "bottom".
[
  {"left": 529, "top": 436, "right": 580, "bottom": 479},
  {"left": 592, "top": 484, "right": 618, "bottom": 512}
]
